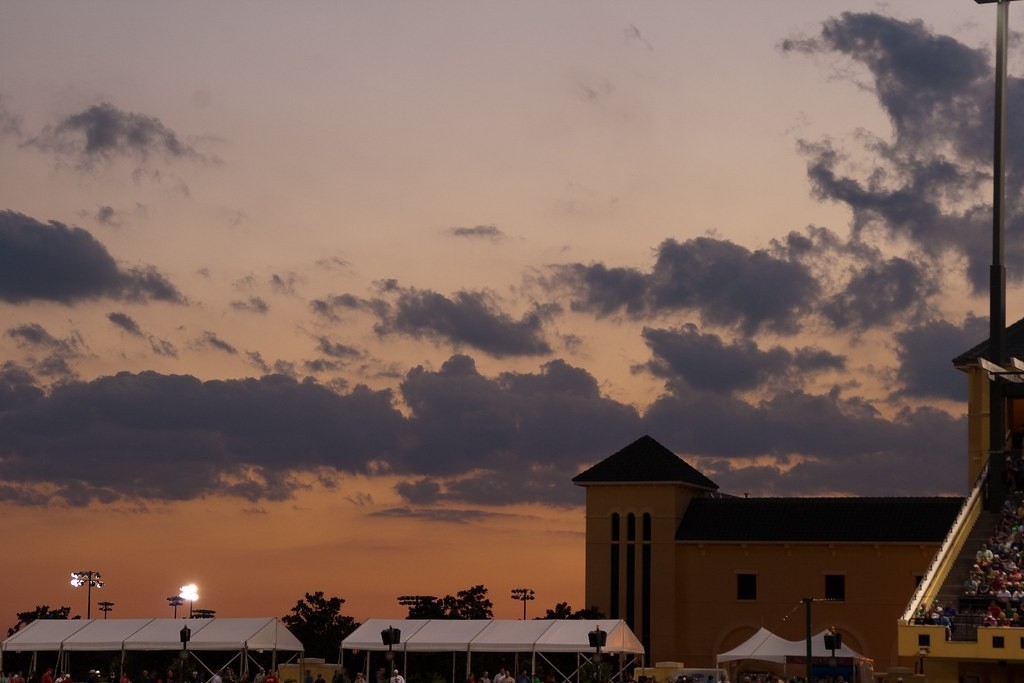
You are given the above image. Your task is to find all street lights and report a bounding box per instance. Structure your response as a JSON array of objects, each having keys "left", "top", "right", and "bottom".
[
  {"left": 98, "top": 602, "right": 114, "bottom": 619},
  {"left": 381, "top": 626, "right": 401, "bottom": 679},
  {"left": 511, "top": 589, "right": 535, "bottom": 620},
  {"left": 71, "top": 571, "right": 104, "bottom": 619},
  {"left": 824, "top": 631, "right": 842, "bottom": 683},
  {"left": 179, "top": 625, "right": 191, "bottom": 683},
  {"left": 588, "top": 624, "right": 607, "bottom": 683},
  {"left": 166, "top": 595, "right": 184, "bottom": 620},
  {"left": 180, "top": 585, "right": 198, "bottom": 619}
]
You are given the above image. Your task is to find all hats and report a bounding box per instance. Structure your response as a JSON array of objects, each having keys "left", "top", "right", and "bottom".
[
  {"left": 394, "top": 670, "right": 398, "bottom": 673},
  {"left": 931, "top": 613, "right": 940, "bottom": 619},
  {"left": 935, "top": 606, "right": 943, "bottom": 612},
  {"left": 931, "top": 600, "right": 939, "bottom": 604},
  {"left": 994, "top": 554, "right": 999, "bottom": 559}
]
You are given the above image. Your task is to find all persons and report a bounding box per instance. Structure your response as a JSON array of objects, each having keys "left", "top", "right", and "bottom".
[
  {"left": 0, "top": 663, "right": 279, "bottom": 683},
  {"left": 478, "top": 671, "right": 491, "bottom": 683},
  {"left": 962, "top": 454, "right": 1024, "bottom": 629},
  {"left": 914, "top": 599, "right": 957, "bottom": 642},
  {"left": 466, "top": 673, "right": 476, "bottom": 683},
  {"left": 492, "top": 668, "right": 555, "bottom": 683},
  {"left": 304, "top": 668, "right": 351, "bottom": 683},
  {"left": 646, "top": 671, "right": 849, "bottom": 683},
  {"left": 376, "top": 667, "right": 405, "bottom": 683},
  {"left": 355, "top": 672, "right": 367, "bottom": 683}
]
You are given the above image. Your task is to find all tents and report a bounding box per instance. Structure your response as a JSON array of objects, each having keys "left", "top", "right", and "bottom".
[
  {"left": 717, "top": 627, "right": 875, "bottom": 683},
  {"left": 340, "top": 619, "right": 646, "bottom": 683},
  {"left": 1, "top": 619, "right": 304, "bottom": 683}
]
